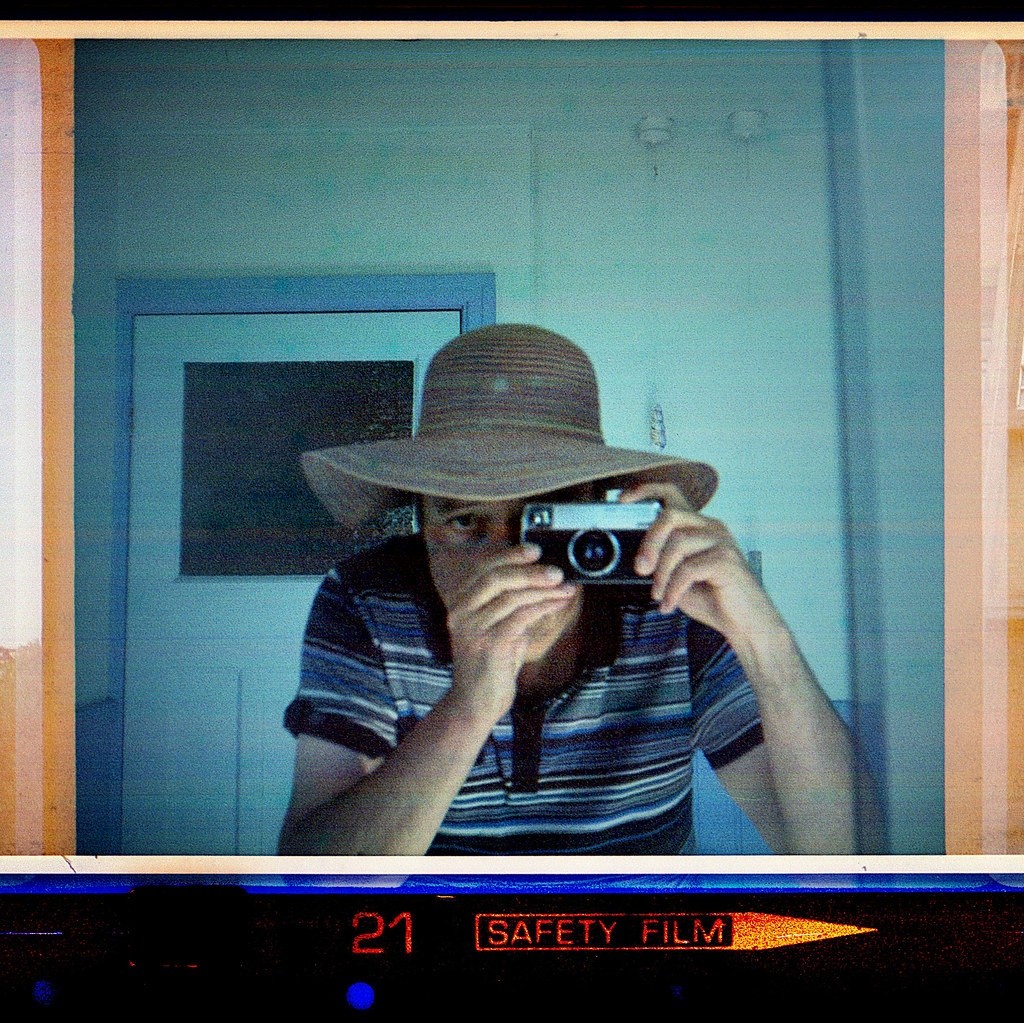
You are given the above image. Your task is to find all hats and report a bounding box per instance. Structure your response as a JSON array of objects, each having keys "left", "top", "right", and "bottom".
[{"left": 298, "top": 324, "right": 718, "bottom": 532}]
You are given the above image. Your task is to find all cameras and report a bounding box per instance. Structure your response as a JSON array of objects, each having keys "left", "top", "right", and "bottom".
[{"left": 520, "top": 488, "right": 664, "bottom": 585}]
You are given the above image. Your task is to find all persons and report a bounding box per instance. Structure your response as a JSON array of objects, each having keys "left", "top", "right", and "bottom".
[{"left": 276, "top": 325, "right": 893, "bottom": 855}]
